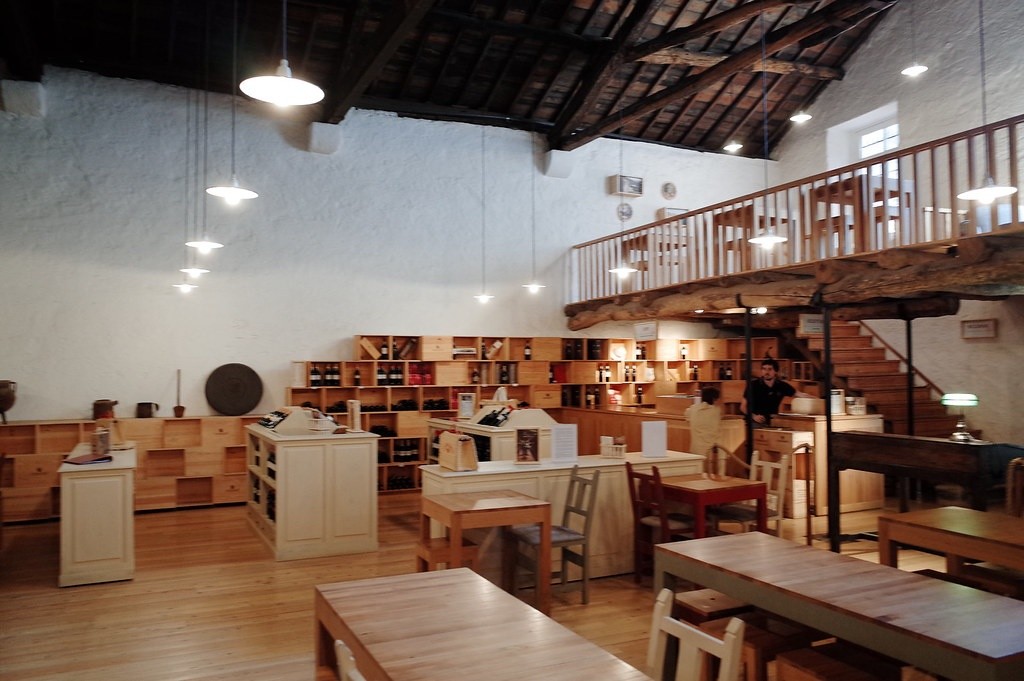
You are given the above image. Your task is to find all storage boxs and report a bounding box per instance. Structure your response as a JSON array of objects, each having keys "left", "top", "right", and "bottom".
[
  {"left": 652, "top": 391, "right": 702, "bottom": 417},
  {"left": 436, "top": 430, "right": 481, "bottom": 473}
]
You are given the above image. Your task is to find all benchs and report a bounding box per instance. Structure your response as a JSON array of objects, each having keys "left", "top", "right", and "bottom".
[
  {"left": 814, "top": 204, "right": 902, "bottom": 235},
  {"left": 725, "top": 232, "right": 752, "bottom": 250}
]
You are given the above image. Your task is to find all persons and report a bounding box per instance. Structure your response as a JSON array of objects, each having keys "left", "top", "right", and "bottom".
[
  {"left": 685, "top": 387, "right": 727, "bottom": 476},
  {"left": 741, "top": 360, "right": 821, "bottom": 481}
]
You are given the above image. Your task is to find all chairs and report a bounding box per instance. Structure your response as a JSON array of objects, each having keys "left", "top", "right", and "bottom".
[
  {"left": 622, "top": 457, "right": 713, "bottom": 583},
  {"left": 714, "top": 449, "right": 790, "bottom": 542},
  {"left": 510, "top": 460, "right": 602, "bottom": 607},
  {"left": 643, "top": 589, "right": 753, "bottom": 681}
]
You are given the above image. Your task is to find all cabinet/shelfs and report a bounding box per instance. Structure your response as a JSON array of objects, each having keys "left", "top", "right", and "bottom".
[
  {"left": 54, "top": 439, "right": 144, "bottom": 591},
  {"left": 0, "top": 414, "right": 259, "bottom": 525},
  {"left": 237, "top": 421, "right": 382, "bottom": 561},
  {"left": 280, "top": 333, "right": 793, "bottom": 496}
]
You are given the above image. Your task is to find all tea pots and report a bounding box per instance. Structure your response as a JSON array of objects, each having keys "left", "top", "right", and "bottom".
[{"left": 90, "top": 399, "right": 119, "bottom": 420}]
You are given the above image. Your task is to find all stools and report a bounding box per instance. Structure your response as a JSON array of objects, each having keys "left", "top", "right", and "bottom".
[{"left": 309, "top": 502, "right": 1024, "bottom": 681}]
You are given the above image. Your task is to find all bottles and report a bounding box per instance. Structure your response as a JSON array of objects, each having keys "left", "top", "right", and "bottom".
[
  {"left": 694, "top": 365, "right": 698, "bottom": 380},
  {"left": 599, "top": 366, "right": 604, "bottom": 381},
  {"left": 683, "top": 346, "right": 685, "bottom": 359},
  {"left": 589, "top": 340, "right": 601, "bottom": 360},
  {"left": 566, "top": 342, "right": 582, "bottom": 360},
  {"left": 524, "top": 341, "right": 531, "bottom": 360},
  {"left": 605, "top": 365, "right": 610, "bottom": 382},
  {"left": 301, "top": 399, "right": 456, "bottom": 490},
  {"left": 726, "top": 363, "right": 732, "bottom": 380},
  {"left": 257, "top": 408, "right": 290, "bottom": 428},
  {"left": 310, "top": 364, "right": 341, "bottom": 386},
  {"left": 587, "top": 389, "right": 591, "bottom": 405},
  {"left": 636, "top": 344, "right": 646, "bottom": 360},
  {"left": 632, "top": 365, "right": 637, "bottom": 381},
  {"left": 638, "top": 386, "right": 642, "bottom": 404},
  {"left": 719, "top": 363, "right": 726, "bottom": 380},
  {"left": 472, "top": 366, "right": 509, "bottom": 384},
  {"left": 562, "top": 389, "right": 567, "bottom": 405},
  {"left": 253, "top": 478, "right": 276, "bottom": 521},
  {"left": 625, "top": 365, "right": 630, "bottom": 381},
  {"left": 594, "top": 388, "right": 600, "bottom": 404},
  {"left": 381, "top": 342, "right": 399, "bottom": 359},
  {"left": 549, "top": 366, "right": 553, "bottom": 383},
  {"left": 377, "top": 365, "right": 402, "bottom": 386},
  {"left": 354, "top": 367, "right": 360, "bottom": 386}
]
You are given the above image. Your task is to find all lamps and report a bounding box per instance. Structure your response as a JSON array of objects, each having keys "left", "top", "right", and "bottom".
[
  {"left": 938, "top": 391, "right": 983, "bottom": 442},
  {"left": 165, "top": 0, "right": 326, "bottom": 297},
  {"left": 472, "top": 0, "right": 1020, "bottom": 307}
]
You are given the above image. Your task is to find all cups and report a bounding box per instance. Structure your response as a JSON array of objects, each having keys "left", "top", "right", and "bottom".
[{"left": 137, "top": 402, "right": 159, "bottom": 418}]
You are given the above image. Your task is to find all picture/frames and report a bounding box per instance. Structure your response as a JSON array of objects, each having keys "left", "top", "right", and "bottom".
[
  {"left": 455, "top": 391, "right": 478, "bottom": 420},
  {"left": 511, "top": 424, "right": 543, "bottom": 467}
]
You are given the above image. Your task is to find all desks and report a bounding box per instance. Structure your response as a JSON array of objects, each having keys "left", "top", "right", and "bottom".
[
  {"left": 829, "top": 429, "right": 998, "bottom": 564},
  {"left": 643, "top": 472, "right": 770, "bottom": 541},
  {"left": 712, "top": 202, "right": 801, "bottom": 274},
  {"left": 875, "top": 504, "right": 1024, "bottom": 600},
  {"left": 621, "top": 232, "right": 691, "bottom": 291},
  {"left": 413, "top": 487, "right": 555, "bottom": 624},
  {"left": 648, "top": 530, "right": 1024, "bottom": 681},
  {"left": 308, "top": 566, "right": 652, "bottom": 681},
  {"left": 811, "top": 173, "right": 917, "bottom": 254}
]
